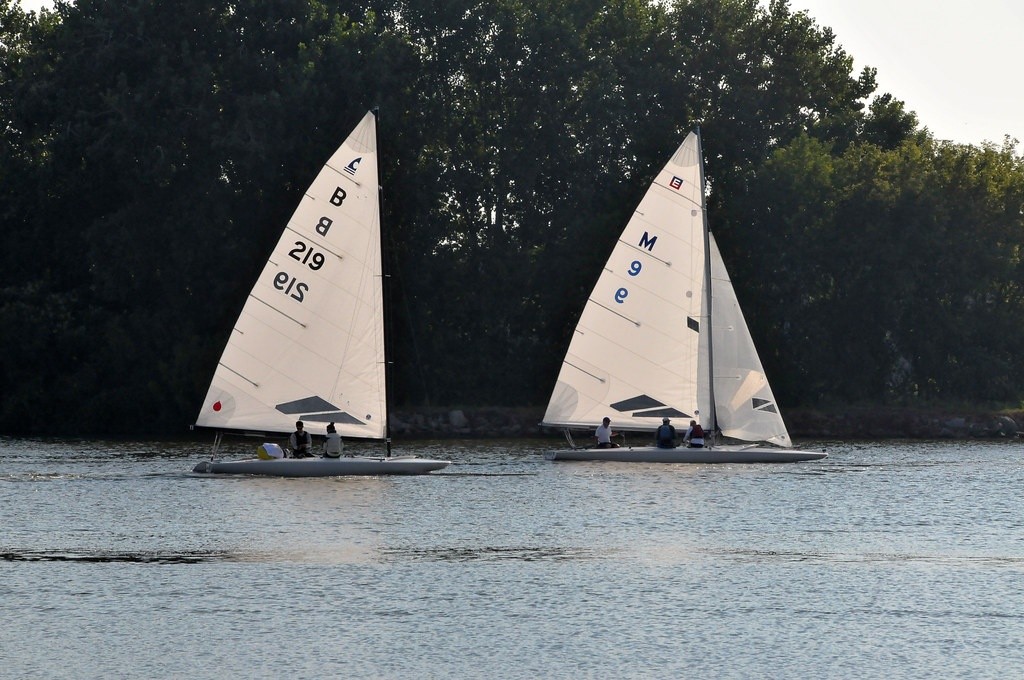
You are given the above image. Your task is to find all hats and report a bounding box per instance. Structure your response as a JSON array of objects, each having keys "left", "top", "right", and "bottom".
[{"left": 662, "top": 417, "right": 671, "bottom": 421}]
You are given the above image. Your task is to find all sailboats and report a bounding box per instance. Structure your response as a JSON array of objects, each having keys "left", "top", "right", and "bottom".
[
  {"left": 536, "top": 127, "right": 835, "bottom": 462},
  {"left": 194, "top": 109, "right": 452, "bottom": 478}
]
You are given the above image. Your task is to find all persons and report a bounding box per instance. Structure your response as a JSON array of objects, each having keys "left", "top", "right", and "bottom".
[
  {"left": 321, "top": 422, "right": 343, "bottom": 458},
  {"left": 656, "top": 417, "right": 675, "bottom": 448},
  {"left": 682, "top": 420, "right": 705, "bottom": 448},
  {"left": 290, "top": 421, "right": 315, "bottom": 458},
  {"left": 596, "top": 417, "right": 620, "bottom": 449}
]
